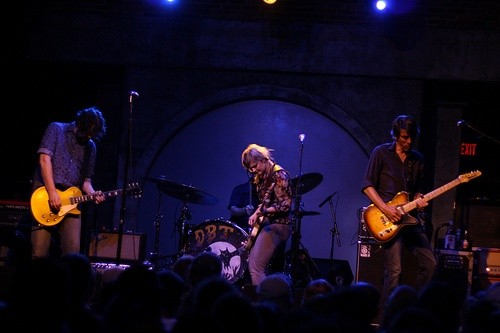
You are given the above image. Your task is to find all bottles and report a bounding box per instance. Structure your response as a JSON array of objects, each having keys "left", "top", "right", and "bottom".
[
  {"left": 444, "top": 218, "right": 456, "bottom": 249},
  {"left": 458, "top": 224, "right": 473, "bottom": 250}
]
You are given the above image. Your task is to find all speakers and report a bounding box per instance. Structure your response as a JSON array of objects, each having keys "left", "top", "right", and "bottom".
[{"left": 86, "top": 263, "right": 142, "bottom": 309}]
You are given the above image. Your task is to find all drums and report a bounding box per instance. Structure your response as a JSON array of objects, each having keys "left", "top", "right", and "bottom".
[{"left": 179, "top": 218, "right": 251, "bottom": 292}]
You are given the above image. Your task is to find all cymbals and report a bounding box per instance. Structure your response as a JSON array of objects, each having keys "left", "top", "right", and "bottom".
[
  {"left": 153, "top": 177, "right": 222, "bottom": 206},
  {"left": 285, "top": 210, "right": 322, "bottom": 218},
  {"left": 290, "top": 172, "right": 324, "bottom": 198}
]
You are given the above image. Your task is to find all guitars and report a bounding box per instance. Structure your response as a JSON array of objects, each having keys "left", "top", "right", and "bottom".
[
  {"left": 245, "top": 181, "right": 276, "bottom": 250},
  {"left": 29, "top": 183, "right": 144, "bottom": 228},
  {"left": 362, "top": 169, "right": 483, "bottom": 244}
]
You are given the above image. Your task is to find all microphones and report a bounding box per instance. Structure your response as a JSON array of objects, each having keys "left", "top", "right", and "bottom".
[
  {"left": 298, "top": 134, "right": 305, "bottom": 150},
  {"left": 457, "top": 121, "right": 474, "bottom": 131},
  {"left": 130, "top": 91, "right": 139, "bottom": 97},
  {"left": 318, "top": 191, "right": 336, "bottom": 208}
]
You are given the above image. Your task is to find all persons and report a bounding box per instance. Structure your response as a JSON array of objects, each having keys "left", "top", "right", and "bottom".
[
  {"left": 0, "top": 241, "right": 500, "bottom": 333},
  {"left": 360, "top": 115, "right": 436, "bottom": 324},
  {"left": 30, "top": 107, "right": 105, "bottom": 257},
  {"left": 241, "top": 144, "right": 296, "bottom": 287}
]
